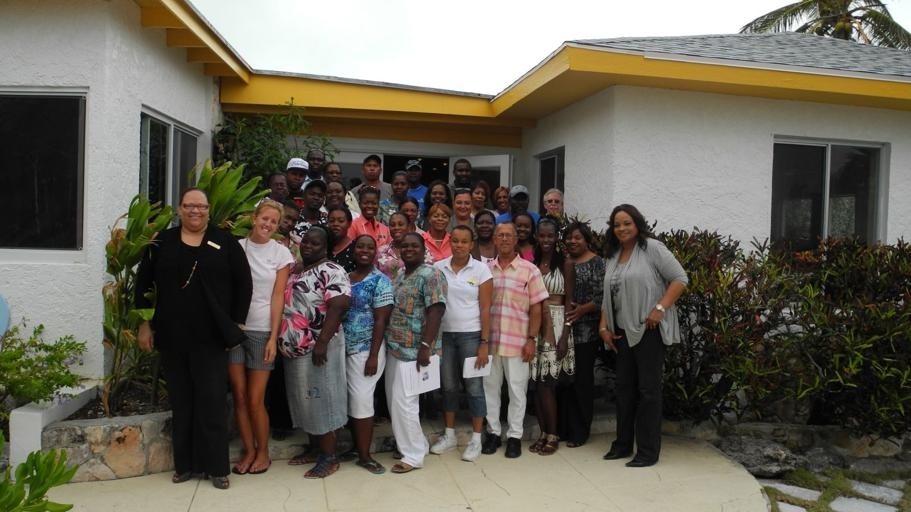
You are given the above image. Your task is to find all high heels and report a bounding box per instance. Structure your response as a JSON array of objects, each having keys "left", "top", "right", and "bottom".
[
  {"left": 173, "top": 470, "right": 193, "bottom": 482},
  {"left": 204, "top": 468, "right": 230, "bottom": 489}
]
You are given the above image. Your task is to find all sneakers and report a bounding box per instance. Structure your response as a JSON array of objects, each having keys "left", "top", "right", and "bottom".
[
  {"left": 462, "top": 439, "right": 483, "bottom": 462},
  {"left": 431, "top": 432, "right": 458, "bottom": 454}
]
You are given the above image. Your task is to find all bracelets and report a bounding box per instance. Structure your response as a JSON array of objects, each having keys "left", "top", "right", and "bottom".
[{"left": 598, "top": 328, "right": 607, "bottom": 333}]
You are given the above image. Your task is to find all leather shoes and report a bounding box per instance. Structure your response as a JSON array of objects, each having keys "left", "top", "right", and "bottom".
[
  {"left": 483, "top": 430, "right": 502, "bottom": 454},
  {"left": 528, "top": 438, "right": 582, "bottom": 457},
  {"left": 604, "top": 451, "right": 619, "bottom": 459},
  {"left": 505, "top": 437, "right": 522, "bottom": 458},
  {"left": 626, "top": 458, "right": 643, "bottom": 466}
]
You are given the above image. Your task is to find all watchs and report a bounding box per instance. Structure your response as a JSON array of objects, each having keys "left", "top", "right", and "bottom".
[{"left": 656, "top": 304, "right": 665, "bottom": 312}]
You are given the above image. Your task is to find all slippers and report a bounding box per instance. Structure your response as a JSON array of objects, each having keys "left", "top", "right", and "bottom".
[
  {"left": 249, "top": 459, "right": 272, "bottom": 472},
  {"left": 340, "top": 449, "right": 416, "bottom": 474},
  {"left": 289, "top": 450, "right": 319, "bottom": 465},
  {"left": 233, "top": 462, "right": 249, "bottom": 474},
  {"left": 305, "top": 461, "right": 340, "bottom": 478}
]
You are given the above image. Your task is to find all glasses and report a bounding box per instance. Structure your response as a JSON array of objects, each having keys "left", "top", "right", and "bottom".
[{"left": 183, "top": 203, "right": 210, "bottom": 210}]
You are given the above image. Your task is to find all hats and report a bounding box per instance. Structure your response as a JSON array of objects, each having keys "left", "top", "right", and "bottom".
[
  {"left": 405, "top": 160, "right": 423, "bottom": 170},
  {"left": 509, "top": 185, "right": 530, "bottom": 199},
  {"left": 304, "top": 179, "right": 328, "bottom": 193},
  {"left": 285, "top": 158, "right": 309, "bottom": 172}
]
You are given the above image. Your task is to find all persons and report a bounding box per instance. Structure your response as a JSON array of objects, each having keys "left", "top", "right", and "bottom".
[
  {"left": 597, "top": 204, "right": 689, "bottom": 468},
  {"left": 226, "top": 149, "right": 607, "bottom": 479},
  {"left": 134, "top": 187, "right": 252, "bottom": 490}
]
